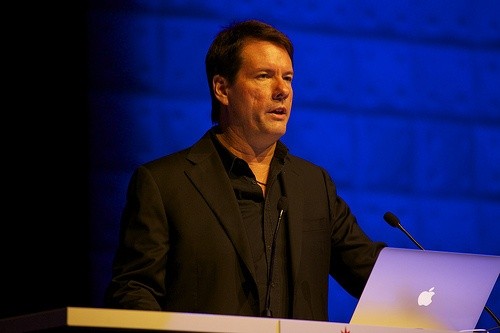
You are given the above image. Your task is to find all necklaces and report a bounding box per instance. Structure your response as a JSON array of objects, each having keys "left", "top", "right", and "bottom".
[{"left": 256, "top": 179, "right": 268, "bottom": 186}]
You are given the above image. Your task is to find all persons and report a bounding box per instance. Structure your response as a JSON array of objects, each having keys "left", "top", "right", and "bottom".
[{"left": 108, "top": 20, "right": 390, "bottom": 333}]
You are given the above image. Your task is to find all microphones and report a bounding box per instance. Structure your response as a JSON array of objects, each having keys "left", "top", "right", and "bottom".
[
  {"left": 262, "top": 196, "right": 290, "bottom": 317},
  {"left": 383, "top": 211, "right": 500, "bottom": 325}
]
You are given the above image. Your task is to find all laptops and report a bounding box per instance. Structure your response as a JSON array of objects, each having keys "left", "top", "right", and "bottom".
[{"left": 348, "top": 247, "right": 500, "bottom": 333}]
